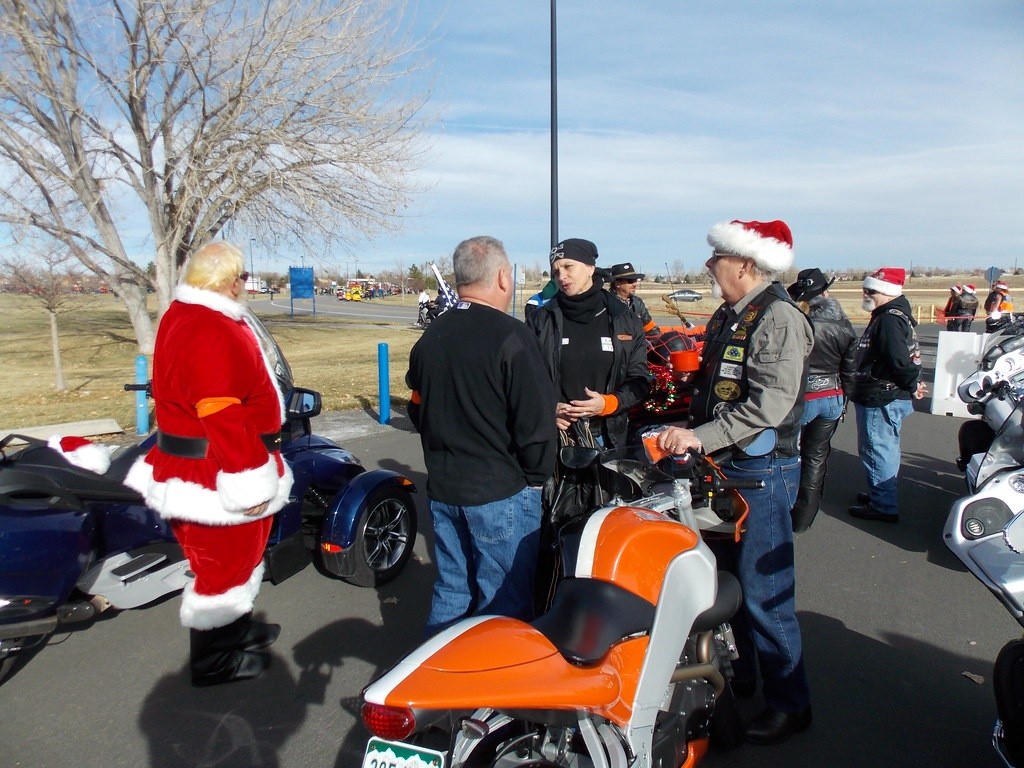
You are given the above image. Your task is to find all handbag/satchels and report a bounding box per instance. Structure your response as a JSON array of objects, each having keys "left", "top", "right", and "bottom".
[{"left": 545, "top": 413, "right": 611, "bottom": 531}]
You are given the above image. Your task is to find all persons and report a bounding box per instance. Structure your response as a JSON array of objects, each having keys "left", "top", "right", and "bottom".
[
  {"left": 404, "top": 236, "right": 560, "bottom": 626},
  {"left": 387, "top": 286, "right": 391, "bottom": 295},
  {"left": 408, "top": 287, "right": 410, "bottom": 294},
  {"left": 786, "top": 268, "right": 859, "bottom": 533},
  {"left": 368, "top": 288, "right": 375, "bottom": 300},
  {"left": 523, "top": 239, "right": 654, "bottom": 519},
  {"left": 413, "top": 289, "right": 430, "bottom": 326},
  {"left": 525, "top": 275, "right": 561, "bottom": 317},
  {"left": 984, "top": 280, "right": 1013, "bottom": 322},
  {"left": 656, "top": 219, "right": 813, "bottom": 745},
  {"left": 847, "top": 267, "right": 930, "bottom": 524},
  {"left": 945, "top": 284, "right": 979, "bottom": 332},
  {"left": 122, "top": 240, "right": 295, "bottom": 687},
  {"left": 610, "top": 262, "right": 661, "bottom": 341}
]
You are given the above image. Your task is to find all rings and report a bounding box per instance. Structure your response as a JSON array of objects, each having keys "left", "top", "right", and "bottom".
[
  {"left": 671, "top": 446, "right": 676, "bottom": 448},
  {"left": 584, "top": 412, "right": 586, "bottom": 416}
]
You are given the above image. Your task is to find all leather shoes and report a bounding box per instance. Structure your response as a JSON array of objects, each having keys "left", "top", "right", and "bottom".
[{"left": 743, "top": 700, "right": 813, "bottom": 746}]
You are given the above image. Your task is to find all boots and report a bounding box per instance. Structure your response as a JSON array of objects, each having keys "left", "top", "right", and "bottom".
[
  {"left": 242, "top": 557, "right": 280, "bottom": 651},
  {"left": 180, "top": 583, "right": 273, "bottom": 688}
]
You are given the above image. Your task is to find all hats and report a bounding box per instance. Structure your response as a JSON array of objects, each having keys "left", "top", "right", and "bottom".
[
  {"left": 787, "top": 268, "right": 835, "bottom": 301},
  {"left": 950, "top": 285, "right": 962, "bottom": 293},
  {"left": 610, "top": 263, "right": 645, "bottom": 279},
  {"left": 963, "top": 284, "right": 976, "bottom": 294},
  {"left": 862, "top": 267, "right": 906, "bottom": 297},
  {"left": 705, "top": 219, "right": 793, "bottom": 273},
  {"left": 995, "top": 281, "right": 1007, "bottom": 289}
]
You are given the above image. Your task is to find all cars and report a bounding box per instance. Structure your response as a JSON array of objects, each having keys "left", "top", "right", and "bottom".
[
  {"left": 261, "top": 286, "right": 274, "bottom": 294},
  {"left": 667, "top": 290, "right": 703, "bottom": 302}
]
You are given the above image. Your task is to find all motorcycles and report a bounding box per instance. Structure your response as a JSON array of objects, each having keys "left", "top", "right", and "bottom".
[
  {"left": 941, "top": 312, "right": 1024, "bottom": 768},
  {"left": 361, "top": 426, "right": 778, "bottom": 768},
  {"left": 0, "top": 375, "right": 418, "bottom": 683},
  {"left": 419, "top": 301, "right": 448, "bottom": 330}
]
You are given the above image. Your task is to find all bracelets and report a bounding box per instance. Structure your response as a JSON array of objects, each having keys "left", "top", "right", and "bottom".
[{"left": 690, "top": 428, "right": 701, "bottom": 454}]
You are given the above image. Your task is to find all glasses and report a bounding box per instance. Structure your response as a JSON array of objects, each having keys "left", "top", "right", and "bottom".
[
  {"left": 864, "top": 288, "right": 880, "bottom": 295},
  {"left": 713, "top": 249, "right": 742, "bottom": 262},
  {"left": 236, "top": 272, "right": 248, "bottom": 281}
]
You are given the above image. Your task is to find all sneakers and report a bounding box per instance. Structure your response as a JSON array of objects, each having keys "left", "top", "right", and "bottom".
[
  {"left": 849, "top": 503, "right": 900, "bottom": 524},
  {"left": 857, "top": 489, "right": 872, "bottom": 504}
]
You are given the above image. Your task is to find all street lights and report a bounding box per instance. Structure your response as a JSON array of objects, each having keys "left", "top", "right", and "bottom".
[
  {"left": 301, "top": 256, "right": 304, "bottom": 268},
  {"left": 250, "top": 238, "right": 256, "bottom": 300},
  {"left": 356, "top": 259, "right": 359, "bottom": 285}
]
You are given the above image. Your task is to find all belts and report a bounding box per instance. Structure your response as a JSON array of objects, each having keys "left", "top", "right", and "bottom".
[{"left": 157, "top": 432, "right": 281, "bottom": 458}]
